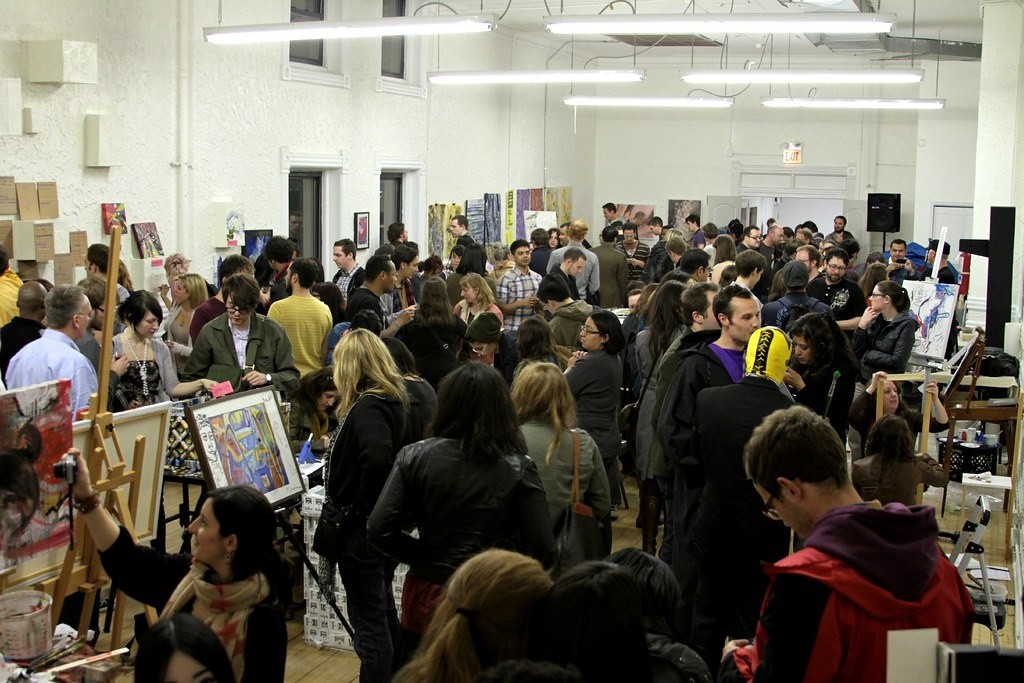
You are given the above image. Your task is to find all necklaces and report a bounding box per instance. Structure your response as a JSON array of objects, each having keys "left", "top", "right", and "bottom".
[
  {"left": 179, "top": 310, "right": 189, "bottom": 327},
  {"left": 124, "top": 328, "right": 148, "bottom": 396}
]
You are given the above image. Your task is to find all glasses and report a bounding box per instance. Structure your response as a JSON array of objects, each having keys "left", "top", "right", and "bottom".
[
  {"left": 828, "top": 264, "right": 845, "bottom": 270},
  {"left": 580, "top": 325, "right": 605, "bottom": 335},
  {"left": 224, "top": 303, "right": 250, "bottom": 315},
  {"left": 703, "top": 267, "right": 713, "bottom": 272},
  {"left": 872, "top": 293, "right": 885, "bottom": 297},
  {"left": 472, "top": 343, "right": 488, "bottom": 354},
  {"left": 761, "top": 475, "right": 801, "bottom": 519},
  {"left": 747, "top": 235, "right": 762, "bottom": 240}
]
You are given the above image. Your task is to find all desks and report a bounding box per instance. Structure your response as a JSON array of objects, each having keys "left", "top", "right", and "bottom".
[
  {"left": 162, "top": 456, "right": 325, "bottom": 622},
  {"left": 959, "top": 472, "right": 1013, "bottom": 561}
]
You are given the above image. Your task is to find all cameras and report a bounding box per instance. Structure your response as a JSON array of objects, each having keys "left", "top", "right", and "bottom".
[{"left": 53, "top": 454, "right": 77, "bottom": 484}]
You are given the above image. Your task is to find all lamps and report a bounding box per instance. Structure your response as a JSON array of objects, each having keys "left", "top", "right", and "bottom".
[
  {"left": 202, "top": 0, "right": 499, "bottom": 46},
  {"left": 563, "top": 34, "right": 735, "bottom": 108},
  {"left": 680, "top": 0, "right": 925, "bottom": 84},
  {"left": 759, "top": 31, "right": 947, "bottom": 110},
  {"left": 426, "top": 0, "right": 648, "bottom": 85},
  {"left": 541, "top": 0, "right": 898, "bottom": 35},
  {"left": 782, "top": 141, "right": 805, "bottom": 165}
]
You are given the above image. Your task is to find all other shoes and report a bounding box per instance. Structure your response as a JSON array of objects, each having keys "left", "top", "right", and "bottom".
[{"left": 610, "top": 504, "right": 618, "bottom": 518}]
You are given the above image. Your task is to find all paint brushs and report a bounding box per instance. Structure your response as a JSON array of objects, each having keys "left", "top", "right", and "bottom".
[{"left": 29, "top": 634, "right": 87, "bottom": 670}]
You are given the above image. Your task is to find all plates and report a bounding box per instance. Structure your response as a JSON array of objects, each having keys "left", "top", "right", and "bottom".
[
  {"left": 960, "top": 443, "right": 980, "bottom": 448},
  {"left": 939, "top": 438, "right": 956, "bottom": 442}
]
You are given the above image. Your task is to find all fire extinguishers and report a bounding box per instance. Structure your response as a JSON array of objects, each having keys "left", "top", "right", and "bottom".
[{"left": 957, "top": 252, "right": 971, "bottom": 294}]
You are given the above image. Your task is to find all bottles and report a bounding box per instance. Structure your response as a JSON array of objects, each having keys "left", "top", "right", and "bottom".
[{"left": 958, "top": 426, "right": 984, "bottom": 445}]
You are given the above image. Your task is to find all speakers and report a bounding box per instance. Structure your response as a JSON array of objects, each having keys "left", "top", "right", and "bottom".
[{"left": 866, "top": 192, "right": 901, "bottom": 233}]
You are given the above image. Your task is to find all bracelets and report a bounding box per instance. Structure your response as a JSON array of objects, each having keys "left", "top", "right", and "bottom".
[
  {"left": 326, "top": 434, "right": 331, "bottom": 438},
  {"left": 536, "top": 298, "right": 539, "bottom": 303},
  {"left": 72, "top": 486, "right": 100, "bottom": 514}
]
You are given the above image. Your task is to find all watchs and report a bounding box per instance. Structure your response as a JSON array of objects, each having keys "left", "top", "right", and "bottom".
[{"left": 265, "top": 372, "right": 272, "bottom": 384}]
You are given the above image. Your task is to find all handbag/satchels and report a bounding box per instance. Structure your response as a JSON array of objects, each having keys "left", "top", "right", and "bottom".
[
  {"left": 618, "top": 401, "right": 639, "bottom": 431},
  {"left": 313, "top": 501, "right": 373, "bottom": 566},
  {"left": 557, "top": 431, "right": 611, "bottom": 571}
]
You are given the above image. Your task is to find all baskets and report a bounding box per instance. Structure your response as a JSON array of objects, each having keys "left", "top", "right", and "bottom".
[{"left": 939, "top": 442, "right": 998, "bottom": 483}]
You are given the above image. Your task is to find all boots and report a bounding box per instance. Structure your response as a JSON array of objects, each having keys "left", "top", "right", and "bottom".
[{"left": 641, "top": 495, "right": 661, "bottom": 556}]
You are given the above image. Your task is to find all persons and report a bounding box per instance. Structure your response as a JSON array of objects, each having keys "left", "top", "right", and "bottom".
[
  {"left": 0, "top": 212, "right": 333, "bottom": 644},
  {"left": 613, "top": 212, "right": 958, "bottom": 683},
  {"left": 131, "top": 614, "right": 243, "bottom": 682},
  {"left": 61, "top": 448, "right": 293, "bottom": 682},
  {"left": 719, "top": 404, "right": 974, "bottom": 683},
  {"left": 310, "top": 203, "right": 621, "bottom": 683}
]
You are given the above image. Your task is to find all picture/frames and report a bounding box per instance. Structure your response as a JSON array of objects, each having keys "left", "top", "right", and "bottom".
[
  {"left": 0, "top": 401, "right": 173, "bottom": 595},
  {"left": 183, "top": 385, "right": 308, "bottom": 510},
  {"left": 354, "top": 212, "right": 369, "bottom": 250}
]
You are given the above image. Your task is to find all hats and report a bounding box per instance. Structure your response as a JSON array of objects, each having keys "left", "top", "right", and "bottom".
[
  {"left": 745, "top": 326, "right": 791, "bottom": 381},
  {"left": 925, "top": 240, "right": 950, "bottom": 255},
  {"left": 466, "top": 312, "right": 501, "bottom": 342},
  {"left": 783, "top": 260, "right": 808, "bottom": 286}
]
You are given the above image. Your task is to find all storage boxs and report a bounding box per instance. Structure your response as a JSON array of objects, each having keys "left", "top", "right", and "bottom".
[{"left": 938, "top": 444, "right": 997, "bottom": 484}]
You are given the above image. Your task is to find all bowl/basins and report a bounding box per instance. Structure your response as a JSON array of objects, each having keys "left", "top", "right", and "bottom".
[{"left": 967, "top": 580, "right": 1007, "bottom": 601}]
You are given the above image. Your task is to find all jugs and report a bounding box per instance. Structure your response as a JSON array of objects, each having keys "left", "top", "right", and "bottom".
[{"left": 985, "top": 422, "right": 1003, "bottom": 444}]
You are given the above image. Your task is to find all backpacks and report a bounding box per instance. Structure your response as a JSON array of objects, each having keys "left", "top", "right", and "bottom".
[{"left": 779, "top": 296, "right": 820, "bottom": 332}]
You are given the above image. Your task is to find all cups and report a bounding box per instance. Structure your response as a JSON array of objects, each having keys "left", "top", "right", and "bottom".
[
  {"left": 985, "top": 434, "right": 997, "bottom": 447},
  {"left": 0, "top": 590, "right": 53, "bottom": 660}
]
